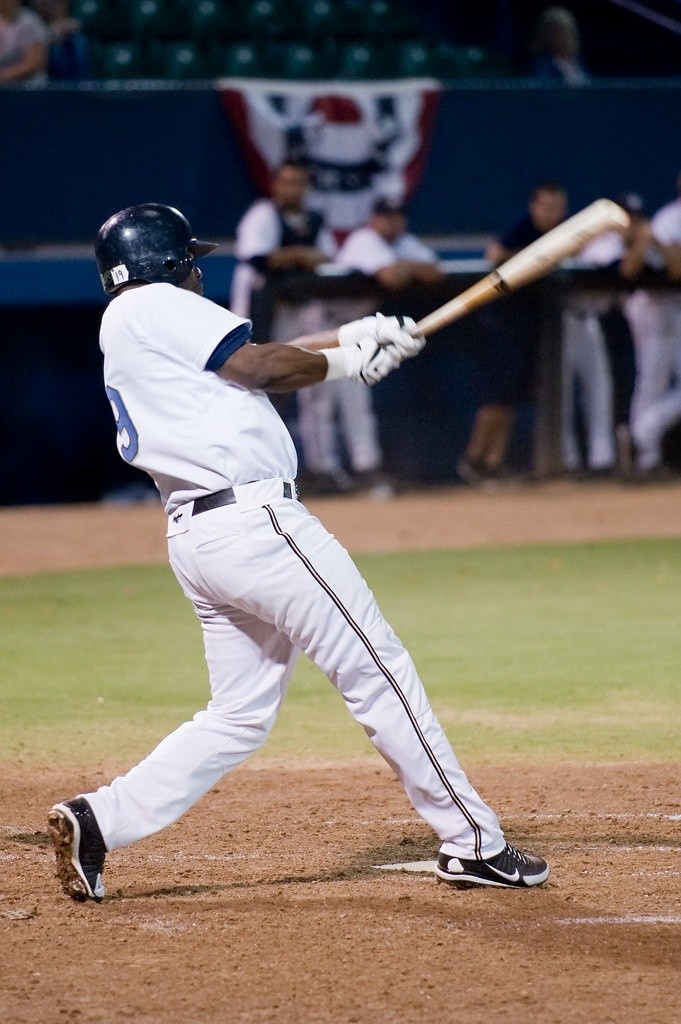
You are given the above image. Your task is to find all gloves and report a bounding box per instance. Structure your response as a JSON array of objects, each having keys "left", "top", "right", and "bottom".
[
  {"left": 360, "top": 310, "right": 428, "bottom": 360},
  {"left": 346, "top": 337, "right": 400, "bottom": 388}
]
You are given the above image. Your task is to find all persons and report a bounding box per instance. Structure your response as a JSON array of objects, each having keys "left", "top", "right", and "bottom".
[
  {"left": 0, "top": 0, "right": 681, "bottom": 492},
  {"left": 46, "top": 203, "right": 550, "bottom": 901}
]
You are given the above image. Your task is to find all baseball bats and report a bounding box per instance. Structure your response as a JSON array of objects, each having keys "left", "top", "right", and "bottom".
[{"left": 403, "top": 199, "right": 629, "bottom": 337}]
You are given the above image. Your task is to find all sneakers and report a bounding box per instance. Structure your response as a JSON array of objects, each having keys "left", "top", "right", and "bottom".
[
  {"left": 435, "top": 839, "right": 550, "bottom": 889},
  {"left": 46, "top": 795, "right": 106, "bottom": 904}
]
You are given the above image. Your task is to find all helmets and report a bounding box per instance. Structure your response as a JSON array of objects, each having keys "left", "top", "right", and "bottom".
[{"left": 95, "top": 202, "right": 217, "bottom": 291}]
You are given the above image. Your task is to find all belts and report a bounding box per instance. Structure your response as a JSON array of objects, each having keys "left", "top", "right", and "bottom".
[{"left": 192, "top": 479, "right": 299, "bottom": 517}]
